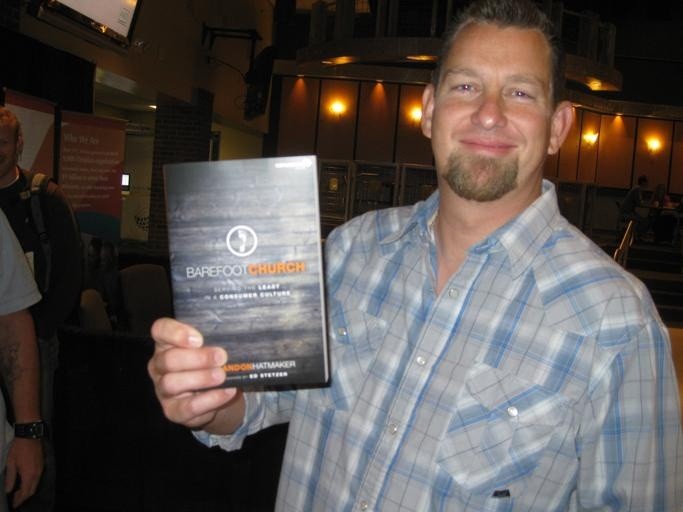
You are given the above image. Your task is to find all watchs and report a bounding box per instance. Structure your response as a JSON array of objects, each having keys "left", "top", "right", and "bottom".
[{"left": 11, "top": 420, "right": 51, "bottom": 441}]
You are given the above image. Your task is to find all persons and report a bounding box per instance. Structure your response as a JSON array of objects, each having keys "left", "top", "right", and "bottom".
[
  {"left": 617, "top": 174, "right": 650, "bottom": 237},
  {"left": 0, "top": 216, "right": 47, "bottom": 506},
  {"left": 2, "top": 110, "right": 86, "bottom": 509},
  {"left": 141, "top": 0, "right": 683, "bottom": 512},
  {"left": 646, "top": 182, "right": 673, "bottom": 216}
]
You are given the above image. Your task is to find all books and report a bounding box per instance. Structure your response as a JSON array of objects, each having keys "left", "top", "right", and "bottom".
[{"left": 161, "top": 152, "right": 333, "bottom": 390}]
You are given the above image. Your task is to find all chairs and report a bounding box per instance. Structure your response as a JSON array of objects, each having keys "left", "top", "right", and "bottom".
[
  {"left": 613, "top": 198, "right": 682, "bottom": 247},
  {"left": 80, "top": 289, "right": 113, "bottom": 333},
  {"left": 117, "top": 262, "right": 173, "bottom": 335}
]
